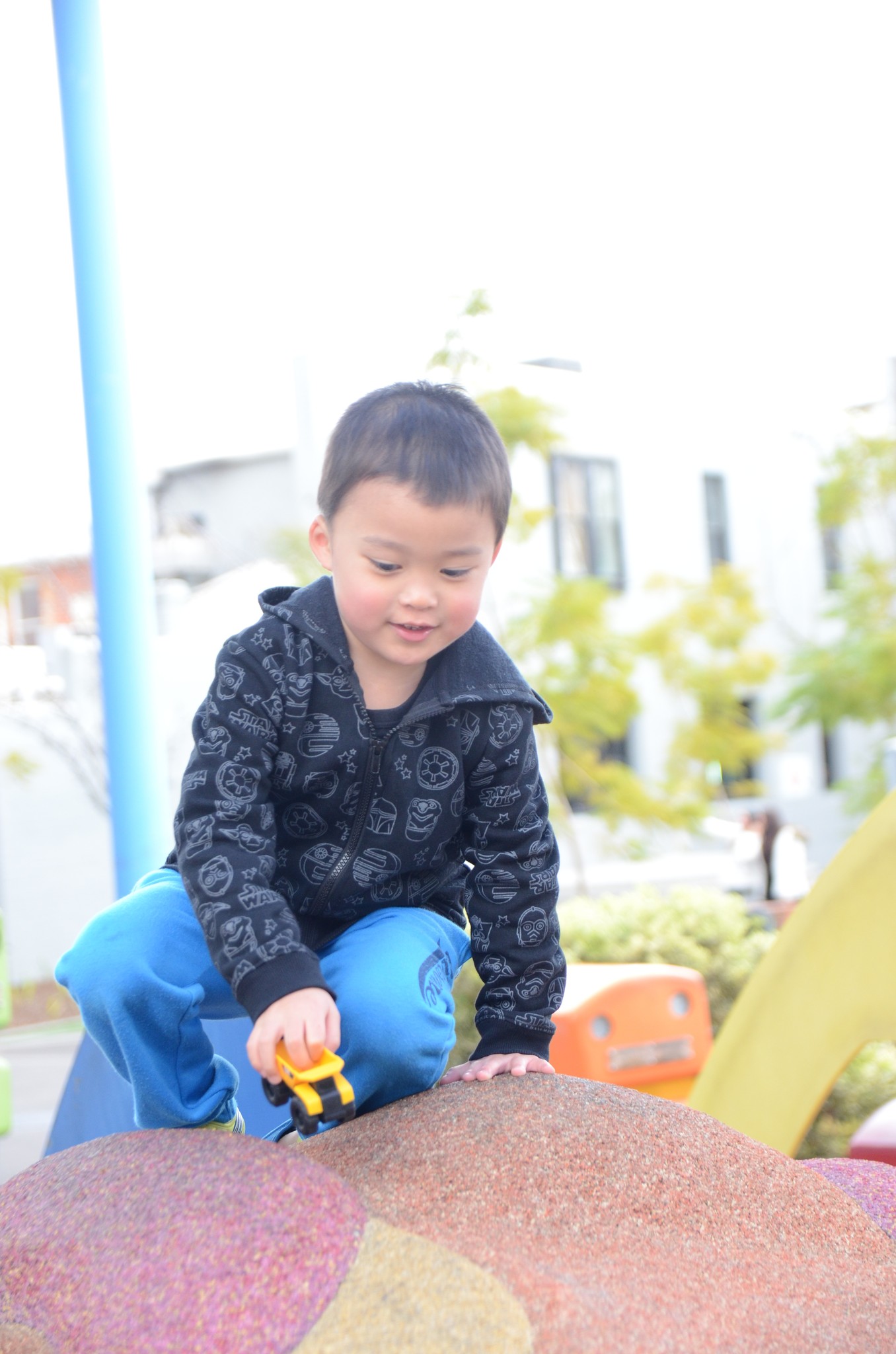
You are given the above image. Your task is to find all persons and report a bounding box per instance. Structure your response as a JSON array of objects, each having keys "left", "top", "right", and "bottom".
[{"left": 51, "top": 380, "right": 568, "bottom": 1144}]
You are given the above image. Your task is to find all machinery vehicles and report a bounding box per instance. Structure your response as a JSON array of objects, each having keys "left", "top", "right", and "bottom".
[{"left": 260, "top": 1037, "right": 359, "bottom": 1137}]
